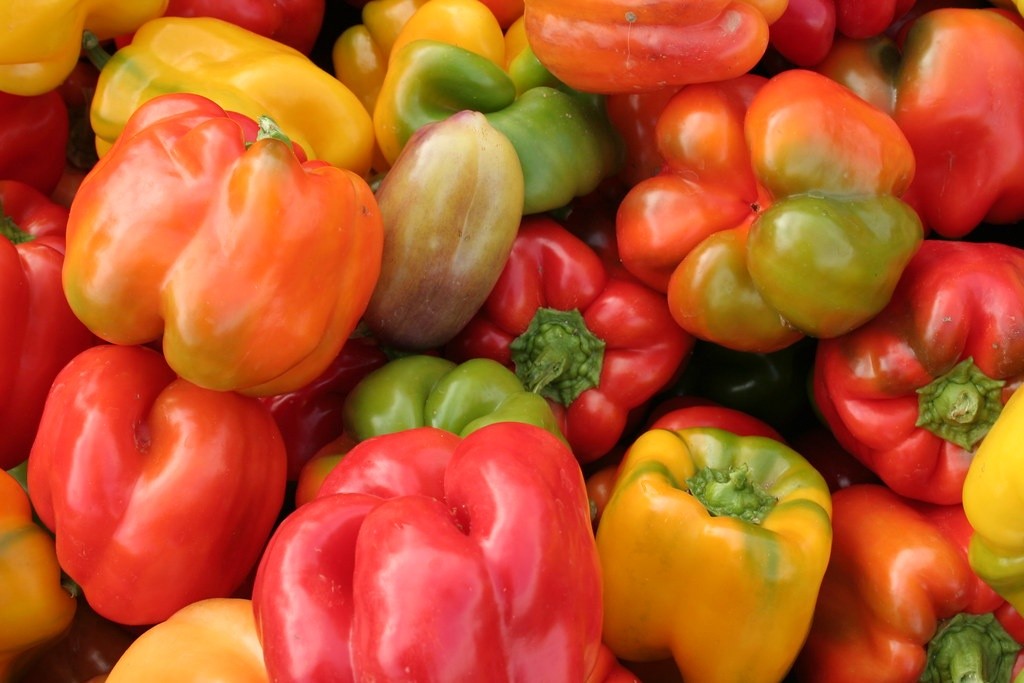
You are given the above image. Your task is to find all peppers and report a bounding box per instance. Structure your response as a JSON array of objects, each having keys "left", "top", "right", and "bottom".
[{"left": 0, "top": 0, "right": 1024, "bottom": 683}]
